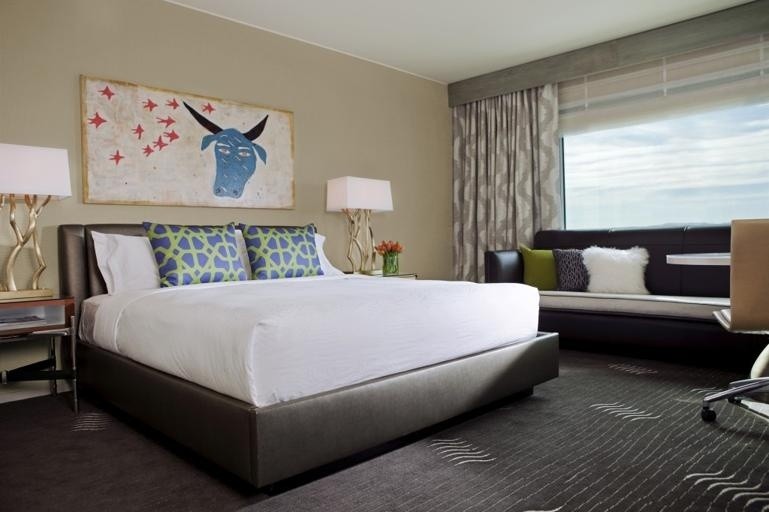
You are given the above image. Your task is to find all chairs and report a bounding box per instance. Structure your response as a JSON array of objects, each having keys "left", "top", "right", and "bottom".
[{"left": 698, "top": 218, "right": 769, "bottom": 424}]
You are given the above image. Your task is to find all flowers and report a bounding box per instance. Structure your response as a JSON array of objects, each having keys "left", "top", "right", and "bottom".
[{"left": 373, "top": 238, "right": 405, "bottom": 276}]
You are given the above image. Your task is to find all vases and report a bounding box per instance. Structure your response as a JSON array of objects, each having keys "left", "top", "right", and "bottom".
[{"left": 381, "top": 253, "right": 396, "bottom": 275}]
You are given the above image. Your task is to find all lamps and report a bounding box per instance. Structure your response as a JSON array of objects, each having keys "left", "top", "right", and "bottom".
[
  {"left": 1, "top": 141, "right": 71, "bottom": 302},
  {"left": 325, "top": 176, "right": 392, "bottom": 275}
]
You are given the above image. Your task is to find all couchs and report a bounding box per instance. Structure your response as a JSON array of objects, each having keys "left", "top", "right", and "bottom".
[{"left": 483, "top": 225, "right": 731, "bottom": 369}]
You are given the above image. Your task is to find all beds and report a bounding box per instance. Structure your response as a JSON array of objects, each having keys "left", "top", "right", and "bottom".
[{"left": 55, "top": 220, "right": 565, "bottom": 501}]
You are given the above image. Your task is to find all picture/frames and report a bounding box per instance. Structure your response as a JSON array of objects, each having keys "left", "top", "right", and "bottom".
[{"left": 81, "top": 76, "right": 296, "bottom": 208}]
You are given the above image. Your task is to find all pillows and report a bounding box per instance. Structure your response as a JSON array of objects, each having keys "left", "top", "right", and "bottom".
[
  {"left": 550, "top": 246, "right": 588, "bottom": 290},
  {"left": 517, "top": 241, "right": 555, "bottom": 288},
  {"left": 315, "top": 233, "right": 345, "bottom": 275},
  {"left": 91, "top": 230, "right": 160, "bottom": 296},
  {"left": 579, "top": 247, "right": 651, "bottom": 296},
  {"left": 238, "top": 221, "right": 329, "bottom": 278},
  {"left": 143, "top": 218, "right": 249, "bottom": 287}
]
